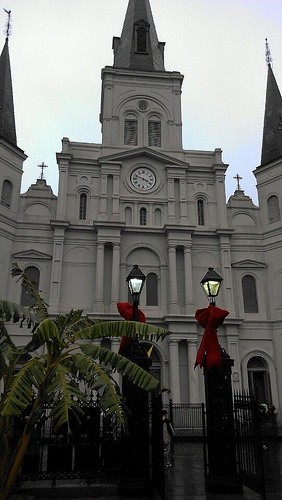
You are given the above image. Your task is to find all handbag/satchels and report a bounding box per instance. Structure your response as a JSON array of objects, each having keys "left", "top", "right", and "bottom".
[
  {"left": 166, "top": 420, "right": 176, "bottom": 436},
  {"left": 273, "top": 406, "right": 280, "bottom": 414}
]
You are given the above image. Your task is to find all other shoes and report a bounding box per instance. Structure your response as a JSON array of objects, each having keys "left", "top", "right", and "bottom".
[{"left": 164, "top": 462, "right": 173, "bottom": 467}]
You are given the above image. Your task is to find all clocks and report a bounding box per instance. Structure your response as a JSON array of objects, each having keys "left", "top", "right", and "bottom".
[{"left": 131, "top": 168, "right": 156, "bottom": 190}]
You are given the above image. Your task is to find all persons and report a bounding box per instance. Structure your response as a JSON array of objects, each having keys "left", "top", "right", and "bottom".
[
  {"left": 160, "top": 409, "right": 176, "bottom": 468},
  {"left": 255, "top": 399, "right": 279, "bottom": 430}
]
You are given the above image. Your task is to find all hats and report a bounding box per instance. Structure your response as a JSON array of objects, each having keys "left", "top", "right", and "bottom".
[{"left": 161, "top": 409, "right": 168, "bottom": 415}]
